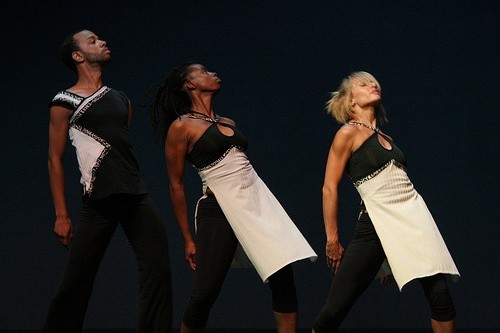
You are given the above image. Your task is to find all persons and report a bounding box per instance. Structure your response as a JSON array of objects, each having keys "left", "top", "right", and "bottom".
[
  {"left": 164, "top": 61, "right": 317, "bottom": 332},
  {"left": 313, "top": 70, "right": 462, "bottom": 333},
  {"left": 40, "top": 28, "right": 172, "bottom": 333}
]
